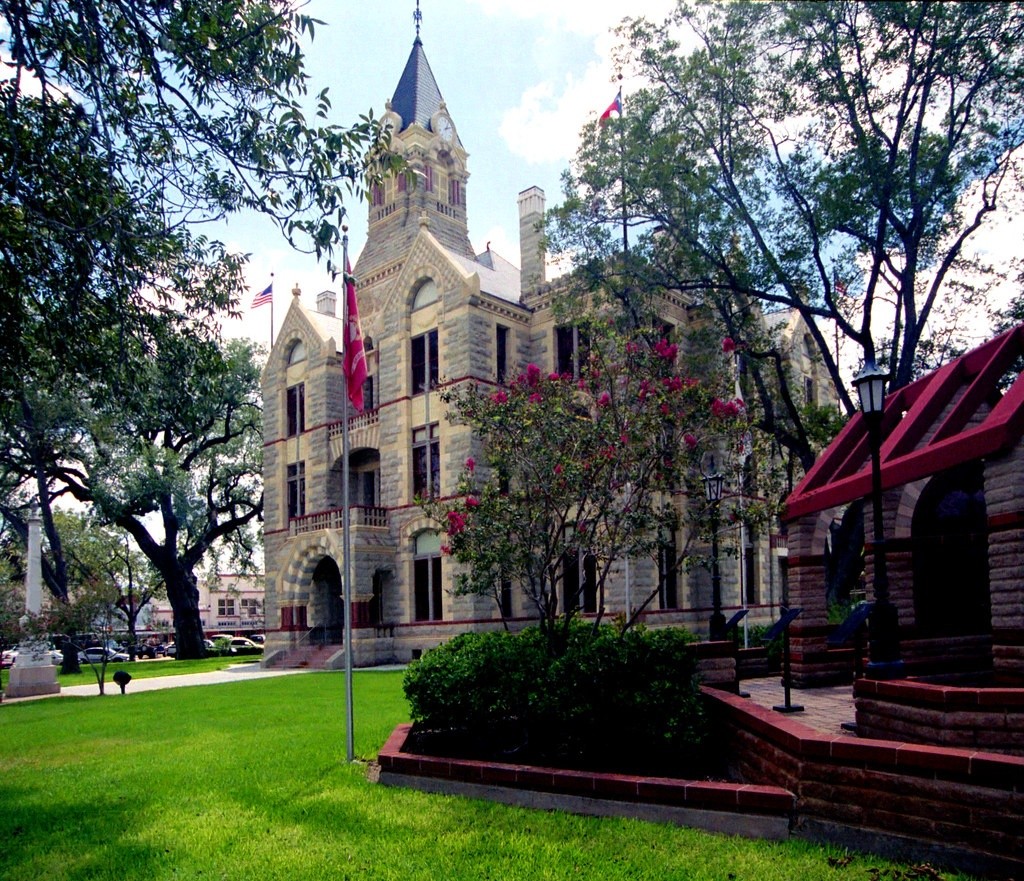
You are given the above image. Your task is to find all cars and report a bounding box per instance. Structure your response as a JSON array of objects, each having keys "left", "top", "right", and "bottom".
[
  {"left": 2, "top": 641, "right": 83, "bottom": 668},
  {"left": 204, "top": 634, "right": 265, "bottom": 650},
  {"left": 78, "top": 647, "right": 130, "bottom": 663},
  {"left": 86, "top": 639, "right": 177, "bottom": 659}
]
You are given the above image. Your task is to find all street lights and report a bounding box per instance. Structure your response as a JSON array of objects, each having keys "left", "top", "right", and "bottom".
[
  {"left": 700, "top": 472, "right": 729, "bottom": 642},
  {"left": 850, "top": 358, "right": 910, "bottom": 681}
]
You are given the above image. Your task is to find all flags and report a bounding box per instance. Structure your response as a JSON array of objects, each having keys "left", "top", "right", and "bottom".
[
  {"left": 251, "top": 283, "right": 273, "bottom": 308},
  {"left": 599, "top": 92, "right": 621, "bottom": 127},
  {"left": 345, "top": 258, "right": 369, "bottom": 414}
]
